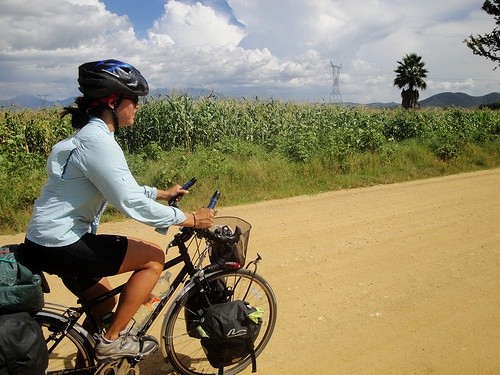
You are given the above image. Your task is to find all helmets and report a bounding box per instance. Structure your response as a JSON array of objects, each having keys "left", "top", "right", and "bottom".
[{"left": 78, "top": 58, "right": 149, "bottom": 96}]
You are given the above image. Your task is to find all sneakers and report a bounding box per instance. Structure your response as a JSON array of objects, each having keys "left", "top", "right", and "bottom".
[{"left": 94, "top": 327, "right": 159, "bottom": 363}]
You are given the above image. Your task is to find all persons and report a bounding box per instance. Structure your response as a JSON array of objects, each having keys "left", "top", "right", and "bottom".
[{"left": 24, "top": 60, "right": 216, "bottom": 375}]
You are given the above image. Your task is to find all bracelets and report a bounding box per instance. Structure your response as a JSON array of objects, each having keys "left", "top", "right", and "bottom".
[{"left": 192, "top": 213, "right": 196, "bottom": 228}]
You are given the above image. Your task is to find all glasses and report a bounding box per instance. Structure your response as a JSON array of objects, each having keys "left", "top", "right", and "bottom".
[{"left": 122, "top": 97, "right": 140, "bottom": 104}]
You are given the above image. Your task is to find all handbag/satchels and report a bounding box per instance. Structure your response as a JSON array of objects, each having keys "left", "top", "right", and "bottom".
[
  {"left": 0, "top": 243, "right": 50, "bottom": 312},
  {"left": 0, "top": 312, "right": 49, "bottom": 375},
  {"left": 200, "top": 299, "right": 263, "bottom": 368},
  {"left": 184, "top": 277, "right": 232, "bottom": 339}
]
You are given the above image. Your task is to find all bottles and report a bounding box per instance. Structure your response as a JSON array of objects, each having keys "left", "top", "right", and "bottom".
[{"left": 129, "top": 272, "right": 172, "bottom": 332}]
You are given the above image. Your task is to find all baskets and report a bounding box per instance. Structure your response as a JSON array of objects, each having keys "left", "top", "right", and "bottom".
[{"left": 205, "top": 216, "right": 251, "bottom": 270}]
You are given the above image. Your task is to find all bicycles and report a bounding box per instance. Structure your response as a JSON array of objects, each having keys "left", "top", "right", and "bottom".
[{"left": 0, "top": 177, "right": 277, "bottom": 375}]
